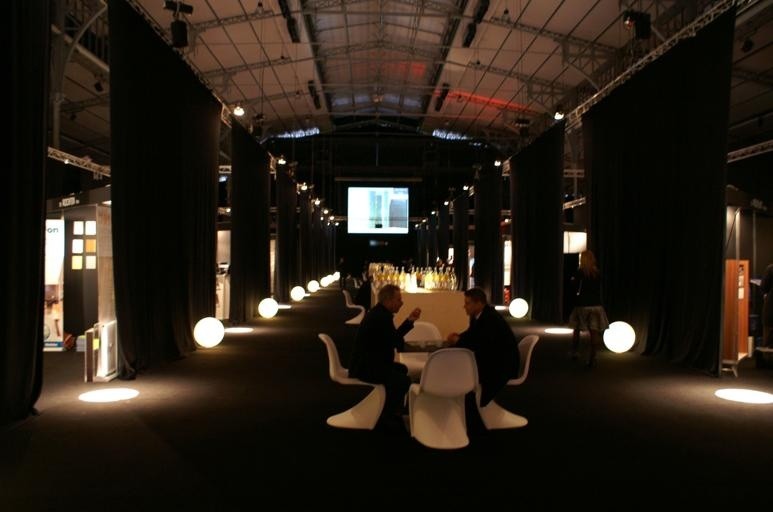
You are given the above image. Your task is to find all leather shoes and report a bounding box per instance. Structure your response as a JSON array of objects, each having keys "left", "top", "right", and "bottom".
[{"left": 567, "top": 350, "right": 598, "bottom": 369}]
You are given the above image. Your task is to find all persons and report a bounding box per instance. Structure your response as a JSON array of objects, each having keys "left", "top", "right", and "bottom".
[
  {"left": 347, "top": 283, "right": 421, "bottom": 422},
  {"left": 566, "top": 250, "right": 610, "bottom": 367},
  {"left": 757, "top": 261, "right": 772, "bottom": 359},
  {"left": 445, "top": 286, "right": 521, "bottom": 408},
  {"left": 335, "top": 255, "right": 349, "bottom": 290}
]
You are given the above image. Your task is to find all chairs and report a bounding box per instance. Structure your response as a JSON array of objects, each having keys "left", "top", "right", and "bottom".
[
  {"left": 343, "top": 290, "right": 366, "bottom": 324},
  {"left": 318, "top": 334, "right": 386, "bottom": 430},
  {"left": 408, "top": 347, "right": 480, "bottom": 449},
  {"left": 400, "top": 321, "right": 441, "bottom": 408},
  {"left": 472, "top": 334, "right": 539, "bottom": 430}
]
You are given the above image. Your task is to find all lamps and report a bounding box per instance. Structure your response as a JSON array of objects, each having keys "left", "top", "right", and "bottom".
[
  {"left": 553, "top": 105, "right": 565, "bottom": 120},
  {"left": 291, "top": 271, "right": 341, "bottom": 302},
  {"left": 193, "top": 317, "right": 225, "bottom": 348},
  {"left": 414, "top": 183, "right": 470, "bottom": 227},
  {"left": 493, "top": 159, "right": 504, "bottom": 167},
  {"left": 301, "top": 182, "right": 340, "bottom": 226},
  {"left": 234, "top": 106, "right": 244, "bottom": 116},
  {"left": 257, "top": 297, "right": 279, "bottom": 319},
  {"left": 602, "top": 321, "right": 636, "bottom": 354},
  {"left": 278, "top": 155, "right": 286, "bottom": 165},
  {"left": 508, "top": 298, "right": 529, "bottom": 319}
]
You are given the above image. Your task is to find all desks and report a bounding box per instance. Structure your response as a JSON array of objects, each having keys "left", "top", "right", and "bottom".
[
  {"left": 397, "top": 341, "right": 463, "bottom": 357},
  {"left": 370, "top": 281, "right": 471, "bottom": 343}
]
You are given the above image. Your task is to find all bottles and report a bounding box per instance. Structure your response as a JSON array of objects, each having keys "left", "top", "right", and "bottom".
[
  {"left": 368, "top": 262, "right": 406, "bottom": 288},
  {"left": 410, "top": 266, "right": 458, "bottom": 291}
]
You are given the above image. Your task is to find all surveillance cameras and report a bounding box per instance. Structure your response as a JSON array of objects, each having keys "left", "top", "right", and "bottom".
[{"left": 94, "top": 77, "right": 107, "bottom": 94}]
